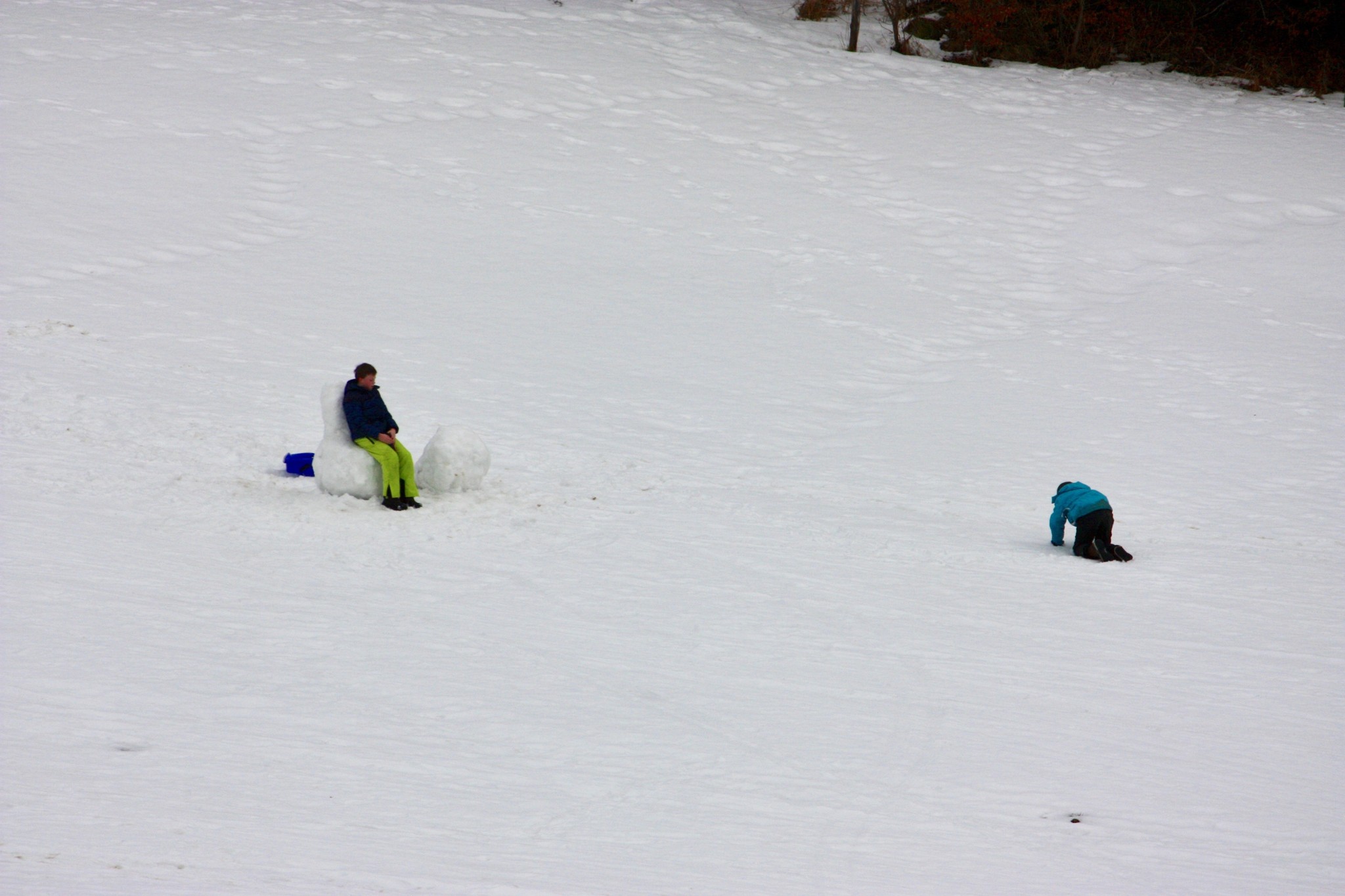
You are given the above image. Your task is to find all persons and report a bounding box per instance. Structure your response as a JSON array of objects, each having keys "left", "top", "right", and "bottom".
[
  {"left": 1050, "top": 480, "right": 1133, "bottom": 563},
  {"left": 341, "top": 363, "right": 423, "bottom": 510}
]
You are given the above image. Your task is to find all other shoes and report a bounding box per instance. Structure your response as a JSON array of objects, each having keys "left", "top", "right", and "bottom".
[
  {"left": 1111, "top": 545, "right": 1133, "bottom": 562},
  {"left": 1092, "top": 540, "right": 1111, "bottom": 562}
]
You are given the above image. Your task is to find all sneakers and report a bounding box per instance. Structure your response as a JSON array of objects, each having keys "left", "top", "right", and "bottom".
[{"left": 381, "top": 496, "right": 423, "bottom": 511}]
